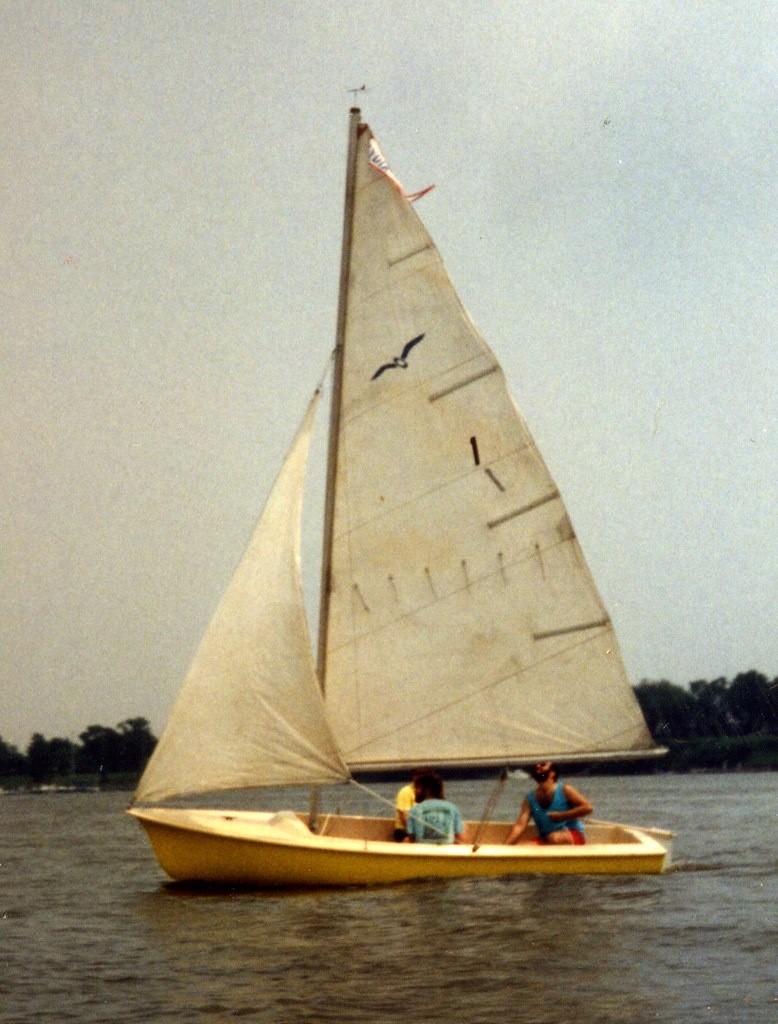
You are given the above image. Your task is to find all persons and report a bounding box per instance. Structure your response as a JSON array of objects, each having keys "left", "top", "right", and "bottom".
[
  {"left": 394, "top": 768, "right": 466, "bottom": 845},
  {"left": 504, "top": 761, "right": 593, "bottom": 845}
]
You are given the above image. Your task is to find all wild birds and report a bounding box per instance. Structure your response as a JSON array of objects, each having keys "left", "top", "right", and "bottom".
[{"left": 370, "top": 333, "right": 426, "bottom": 379}]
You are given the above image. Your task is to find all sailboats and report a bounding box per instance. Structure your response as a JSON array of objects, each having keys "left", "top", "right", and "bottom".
[{"left": 123, "top": 97, "right": 679, "bottom": 891}]
